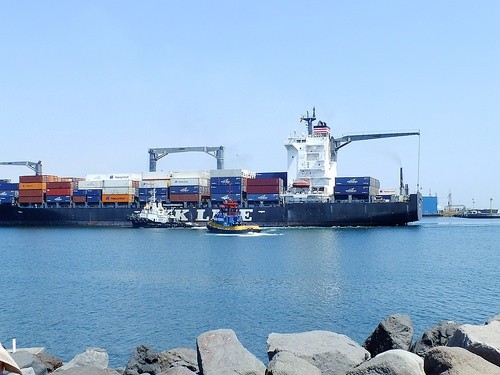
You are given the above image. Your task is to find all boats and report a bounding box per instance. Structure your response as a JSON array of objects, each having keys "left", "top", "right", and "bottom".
[
  {"left": 130, "top": 184, "right": 189, "bottom": 228},
  {"left": 204, "top": 200, "right": 262, "bottom": 233}
]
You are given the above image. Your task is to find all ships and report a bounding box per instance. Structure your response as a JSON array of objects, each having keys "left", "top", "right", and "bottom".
[{"left": 0, "top": 108, "right": 423, "bottom": 226}]
